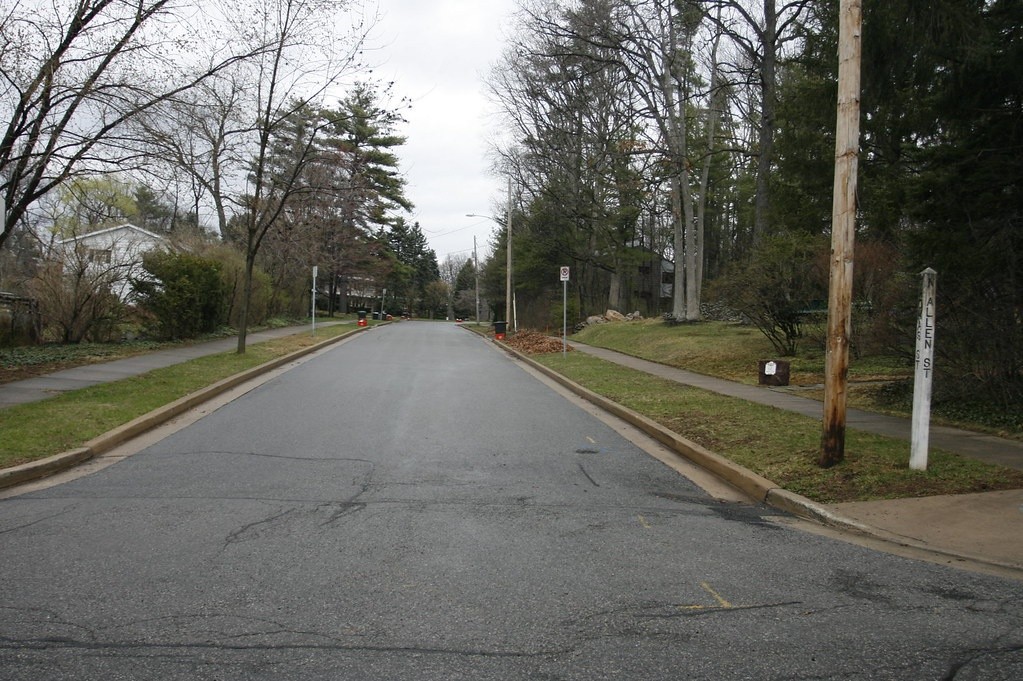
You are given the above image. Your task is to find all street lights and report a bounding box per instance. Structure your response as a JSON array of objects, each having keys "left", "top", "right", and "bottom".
[{"left": 466, "top": 214, "right": 511, "bottom": 333}]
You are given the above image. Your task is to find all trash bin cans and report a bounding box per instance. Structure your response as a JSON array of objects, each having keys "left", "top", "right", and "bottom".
[
  {"left": 446, "top": 317, "right": 450, "bottom": 321},
  {"left": 494, "top": 320, "right": 508, "bottom": 340},
  {"left": 372, "top": 312, "right": 379, "bottom": 319},
  {"left": 382, "top": 313, "right": 386, "bottom": 320},
  {"left": 358, "top": 311, "right": 367, "bottom": 325},
  {"left": 386, "top": 315, "right": 393, "bottom": 320}
]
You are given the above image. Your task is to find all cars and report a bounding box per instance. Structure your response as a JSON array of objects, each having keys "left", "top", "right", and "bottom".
[
  {"left": 464, "top": 317, "right": 470, "bottom": 321},
  {"left": 457, "top": 318, "right": 463, "bottom": 322}
]
